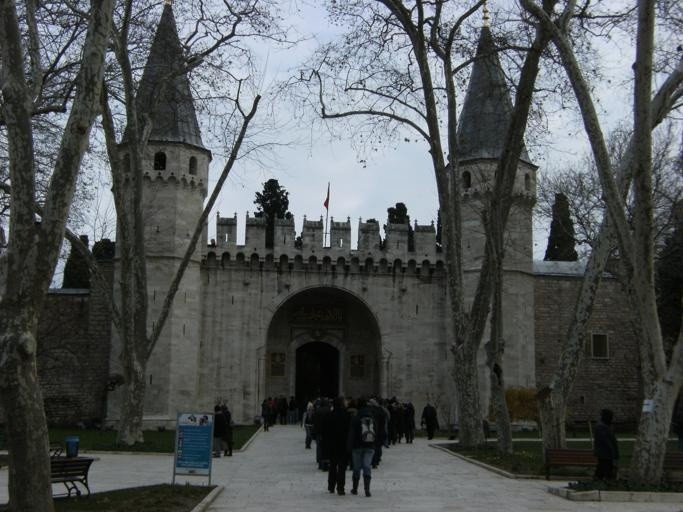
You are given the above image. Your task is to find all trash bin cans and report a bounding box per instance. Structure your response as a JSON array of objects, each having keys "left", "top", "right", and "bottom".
[{"left": 64, "top": 436, "right": 80, "bottom": 457}]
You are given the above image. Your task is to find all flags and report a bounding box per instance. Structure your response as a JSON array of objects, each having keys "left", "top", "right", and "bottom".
[{"left": 323, "top": 184, "right": 330, "bottom": 209}]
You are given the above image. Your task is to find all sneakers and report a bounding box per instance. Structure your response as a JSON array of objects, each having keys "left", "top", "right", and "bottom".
[
  {"left": 337, "top": 489, "right": 345, "bottom": 496},
  {"left": 329, "top": 489, "right": 335, "bottom": 494}
]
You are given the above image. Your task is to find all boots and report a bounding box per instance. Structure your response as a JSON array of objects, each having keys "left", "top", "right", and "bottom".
[
  {"left": 351, "top": 476, "right": 360, "bottom": 496},
  {"left": 363, "top": 475, "right": 372, "bottom": 498}
]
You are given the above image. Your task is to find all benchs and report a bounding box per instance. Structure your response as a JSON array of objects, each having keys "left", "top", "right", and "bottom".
[
  {"left": 537, "top": 446, "right": 600, "bottom": 482},
  {"left": 47, "top": 455, "right": 94, "bottom": 499},
  {"left": 660, "top": 452, "right": 683, "bottom": 480}
]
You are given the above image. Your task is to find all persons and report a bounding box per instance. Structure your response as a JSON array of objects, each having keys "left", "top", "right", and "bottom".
[
  {"left": 592, "top": 407, "right": 620, "bottom": 484},
  {"left": 318, "top": 392, "right": 360, "bottom": 495},
  {"left": 219, "top": 404, "right": 234, "bottom": 456},
  {"left": 260, "top": 393, "right": 298, "bottom": 431},
  {"left": 298, "top": 391, "right": 413, "bottom": 469},
  {"left": 211, "top": 404, "right": 223, "bottom": 458},
  {"left": 419, "top": 401, "right": 438, "bottom": 440},
  {"left": 348, "top": 397, "right": 384, "bottom": 497}
]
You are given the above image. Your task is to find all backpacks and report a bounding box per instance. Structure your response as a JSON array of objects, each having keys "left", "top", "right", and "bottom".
[{"left": 358, "top": 415, "right": 378, "bottom": 444}]
